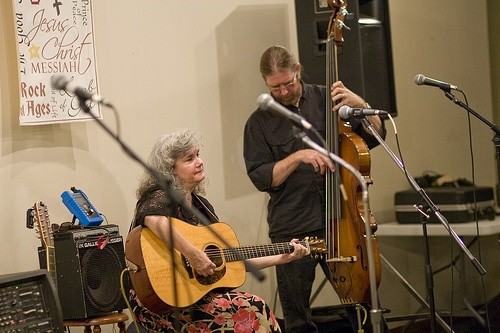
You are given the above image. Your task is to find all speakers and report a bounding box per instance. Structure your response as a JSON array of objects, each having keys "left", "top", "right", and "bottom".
[
  {"left": 294, "top": 1, "right": 397, "bottom": 118},
  {"left": 54, "top": 224, "right": 132, "bottom": 321},
  {"left": 310, "top": 302, "right": 355, "bottom": 333}
]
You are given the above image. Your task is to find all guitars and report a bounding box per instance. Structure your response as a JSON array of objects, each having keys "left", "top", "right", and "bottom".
[
  {"left": 26, "top": 201, "right": 57, "bottom": 289},
  {"left": 124, "top": 216, "right": 327, "bottom": 314}
]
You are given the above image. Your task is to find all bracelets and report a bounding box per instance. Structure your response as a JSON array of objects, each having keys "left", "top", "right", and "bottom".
[{"left": 366, "top": 103, "right": 372, "bottom": 109}]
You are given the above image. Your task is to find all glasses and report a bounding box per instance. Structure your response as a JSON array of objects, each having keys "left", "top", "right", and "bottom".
[{"left": 266, "top": 72, "right": 297, "bottom": 92}]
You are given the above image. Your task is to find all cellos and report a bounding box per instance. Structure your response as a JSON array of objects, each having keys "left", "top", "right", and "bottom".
[{"left": 326, "top": 0, "right": 382, "bottom": 333}]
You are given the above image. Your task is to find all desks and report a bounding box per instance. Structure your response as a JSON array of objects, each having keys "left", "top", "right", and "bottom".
[{"left": 371, "top": 216, "right": 500, "bottom": 333}]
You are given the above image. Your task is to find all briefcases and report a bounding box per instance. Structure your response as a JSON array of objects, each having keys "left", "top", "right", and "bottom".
[{"left": 393, "top": 184, "right": 495, "bottom": 224}]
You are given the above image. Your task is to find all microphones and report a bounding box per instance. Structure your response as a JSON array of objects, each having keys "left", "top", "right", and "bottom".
[
  {"left": 49, "top": 75, "right": 104, "bottom": 105},
  {"left": 256, "top": 94, "right": 313, "bottom": 128},
  {"left": 414, "top": 74, "right": 457, "bottom": 90},
  {"left": 338, "top": 105, "right": 389, "bottom": 119}
]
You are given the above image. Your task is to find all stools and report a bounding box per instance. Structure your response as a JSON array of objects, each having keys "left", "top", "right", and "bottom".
[{"left": 63, "top": 312, "right": 129, "bottom": 333}]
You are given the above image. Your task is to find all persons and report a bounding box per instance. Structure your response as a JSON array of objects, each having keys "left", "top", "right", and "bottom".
[
  {"left": 128, "top": 131, "right": 281, "bottom": 333},
  {"left": 243, "top": 46, "right": 387, "bottom": 333}
]
